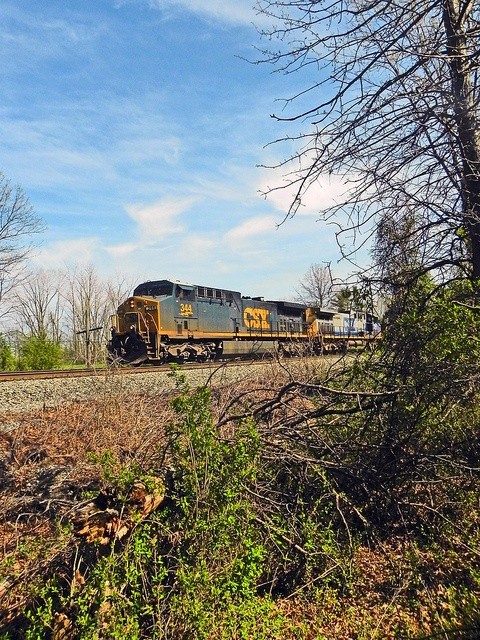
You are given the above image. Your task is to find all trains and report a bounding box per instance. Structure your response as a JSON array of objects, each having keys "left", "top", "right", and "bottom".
[{"left": 106, "top": 279, "right": 383, "bottom": 367}]
